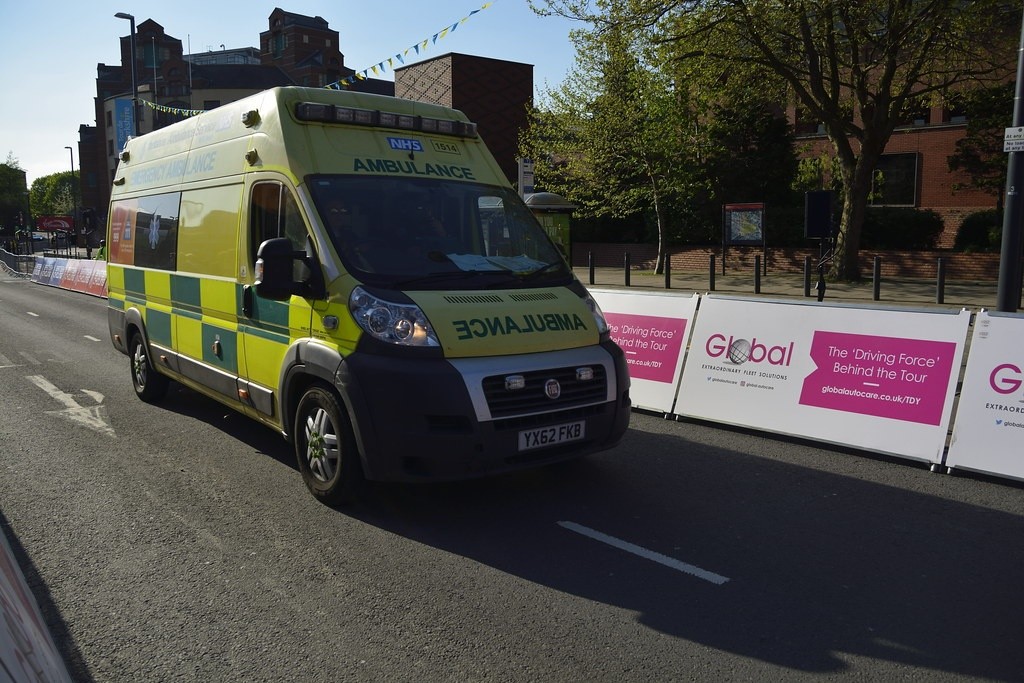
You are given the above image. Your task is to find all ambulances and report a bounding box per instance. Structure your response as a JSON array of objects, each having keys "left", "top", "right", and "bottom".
[{"left": 102, "top": 86, "right": 633, "bottom": 510}]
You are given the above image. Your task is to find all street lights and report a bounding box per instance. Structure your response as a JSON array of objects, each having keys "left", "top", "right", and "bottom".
[
  {"left": 113, "top": 12, "right": 141, "bottom": 139},
  {"left": 22, "top": 191, "right": 35, "bottom": 255},
  {"left": 64, "top": 146, "right": 79, "bottom": 260}
]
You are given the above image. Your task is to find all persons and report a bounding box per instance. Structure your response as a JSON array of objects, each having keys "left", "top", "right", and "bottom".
[{"left": 93, "top": 240, "right": 106, "bottom": 261}]
